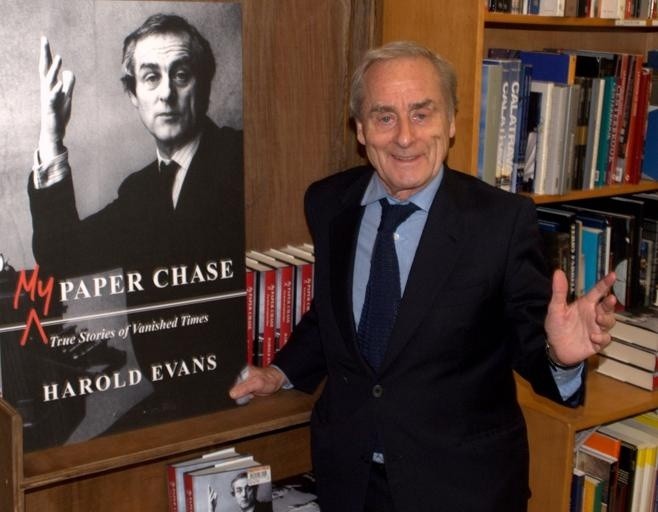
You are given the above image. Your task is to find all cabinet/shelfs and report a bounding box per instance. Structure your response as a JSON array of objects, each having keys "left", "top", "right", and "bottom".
[
  {"left": 381, "top": 0, "right": 658, "bottom": 512},
  {"left": 1, "top": 374, "right": 334, "bottom": 512}
]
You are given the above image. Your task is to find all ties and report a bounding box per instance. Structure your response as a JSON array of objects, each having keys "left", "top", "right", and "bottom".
[
  {"left": 158, "top": 161, "right": 178, "bottom": 203},
  {"left": 358, "top": 198, "right": 419, "bottom": 369}
]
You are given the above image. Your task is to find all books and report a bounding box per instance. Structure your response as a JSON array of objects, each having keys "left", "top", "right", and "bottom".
[
  {"left": 246, "top": 243, "right": 315, "bottom": 367},
  {"left": 168, "top": 448, "right": 320, "bottom": 512}
]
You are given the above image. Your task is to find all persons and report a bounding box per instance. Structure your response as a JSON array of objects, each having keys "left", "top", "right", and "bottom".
[
  {"left": 27, "top": 14, "right": 246, "bottom": 437},
  {"left": 228, "top": 39, "right": 616, "bottom": 512}
]
